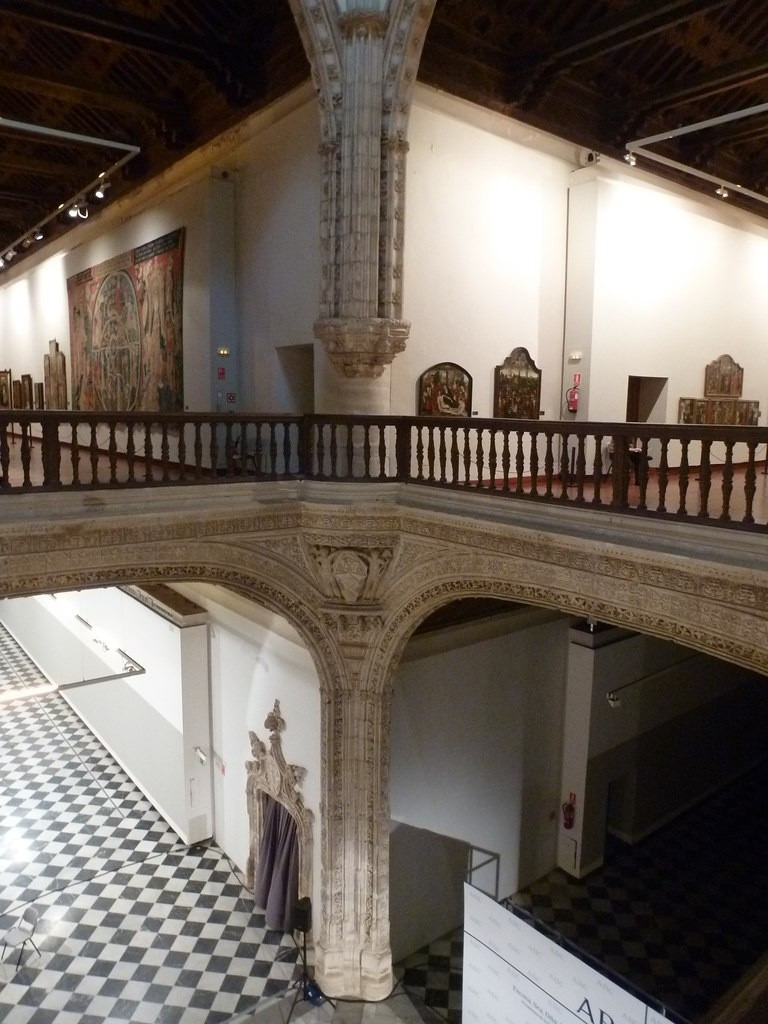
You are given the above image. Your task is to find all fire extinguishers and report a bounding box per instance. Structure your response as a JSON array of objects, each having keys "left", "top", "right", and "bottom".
[
  {"left": 561, "top": 801, "right": 575, "bottom": 830},
  {"left": 566, "top": 384, "right": 580, "bottom": 412}
]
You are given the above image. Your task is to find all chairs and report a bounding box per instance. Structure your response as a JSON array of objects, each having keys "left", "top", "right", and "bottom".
[{"left": 2, "top": 907, "right": 41, "bottom": 971}]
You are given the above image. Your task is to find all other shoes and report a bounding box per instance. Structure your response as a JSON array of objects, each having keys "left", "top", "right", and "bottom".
[
  {"left": 647, "top": 456, "right": 653, "bottom": 461},
  {"left": 635, "top": 481, "right": 640, "bottom": 485}
]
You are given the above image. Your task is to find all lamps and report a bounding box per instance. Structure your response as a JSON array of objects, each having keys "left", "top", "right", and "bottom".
[
  {"left": 35, "top": 226, "right": 43, "bottom": 240},
  {"left": 4, "top": 248, "right": 17, "bottom": 261},
  {"left": 95, "top": 178, "right": 112, "bottom": 198},
  {"left": 23, "top": 237, "right": 31, "bottom": 248},
  {"left": 69, "top": 196, "right": 89, "bottom": 219}
]
